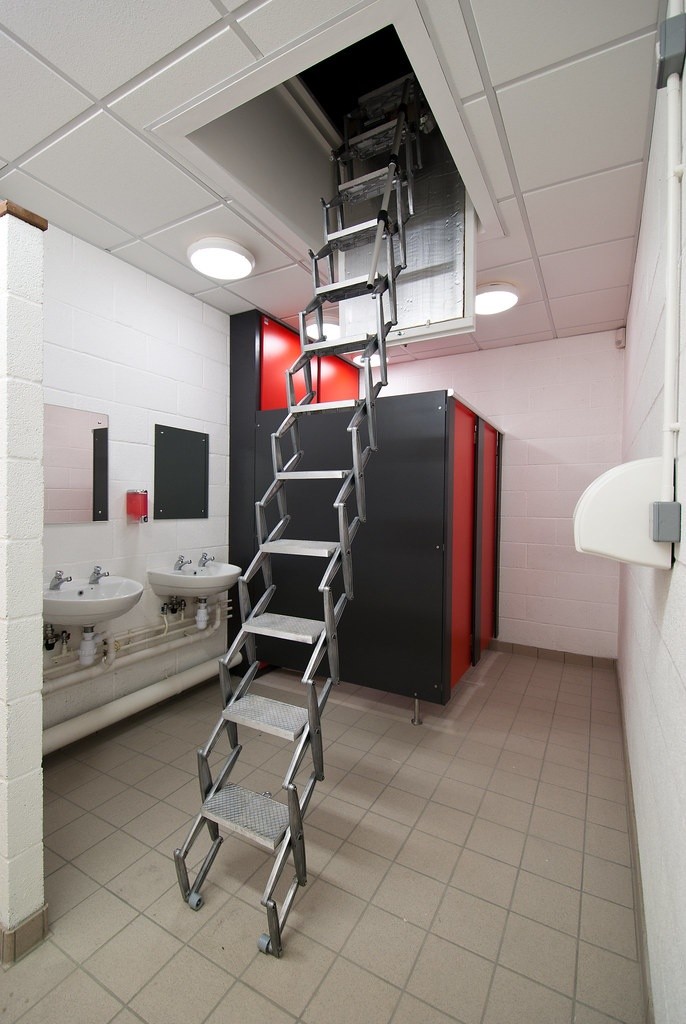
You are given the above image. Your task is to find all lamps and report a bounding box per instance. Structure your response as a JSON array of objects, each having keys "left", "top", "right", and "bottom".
[
  {"left": 303, "top": 313, "right": 341, "bottom": 341},
  {"left": 473, "top": 282, "right": 521, "bottom": 318},
  {"left": 187, "top": 237, "right": 255, "bottom": 283}
]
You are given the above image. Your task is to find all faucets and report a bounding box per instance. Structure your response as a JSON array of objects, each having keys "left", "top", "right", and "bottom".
[
  {"left": 88, "top": 565, "right": 110, "bottom": 584},
  {"left": 173, "top": 555, "right": 192, "bottom": 571},
  {"left": 49, "top": 570, "right": 73, "bottom": 591},
  {"left": 197, "top": 552, "right": 216, "bottom": 567}
]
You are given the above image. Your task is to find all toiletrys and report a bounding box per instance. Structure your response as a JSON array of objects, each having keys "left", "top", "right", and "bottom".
[{"left": 126, "top": 488, "right": 149, "bottom": 526}]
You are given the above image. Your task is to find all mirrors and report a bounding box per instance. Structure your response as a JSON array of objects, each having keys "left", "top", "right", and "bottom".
[{"left": 42, "top": 403, "right": 109, "bottom": 525}]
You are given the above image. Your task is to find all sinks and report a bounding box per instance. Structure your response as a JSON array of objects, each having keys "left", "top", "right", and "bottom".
[
  {"left": 42, "top": 575, "right": 145, "bottom": 627},
  {"left": 146, "top": 561, "right": 243, "bottom": 599}
]
